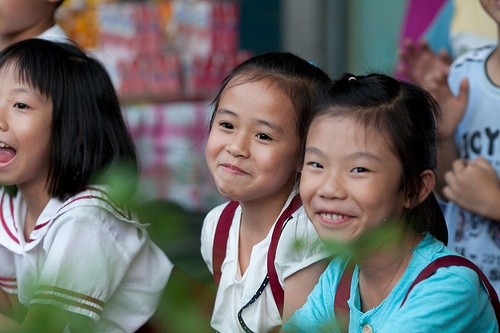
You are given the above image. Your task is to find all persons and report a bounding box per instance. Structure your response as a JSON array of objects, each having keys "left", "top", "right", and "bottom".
[
  {"left": 279, "top": 73, "right": 500, "bottom": 333},
  {"left": 0, "top": 0, "right": 75, "bottom": 51},
  {"left": 422, "top": 0, "right": 500, "bottom": 302},
  {"left": 397, "top": 0, "right": 496, "bottom": 91},
  {"left": 200, "top": 53, "right": 334, "bottom": 333},
  {"left": 0, "top": 38, "right": 174, "bottom": 333}
]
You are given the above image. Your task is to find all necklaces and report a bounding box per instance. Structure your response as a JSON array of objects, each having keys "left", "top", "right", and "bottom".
[{"left": 360, "top": 225, "right": 413, "bottom": 306}]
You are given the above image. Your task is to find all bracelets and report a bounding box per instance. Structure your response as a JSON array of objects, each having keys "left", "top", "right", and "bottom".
[{"left": 436, "top": 138, "right": 455, "bottom": 146}]
